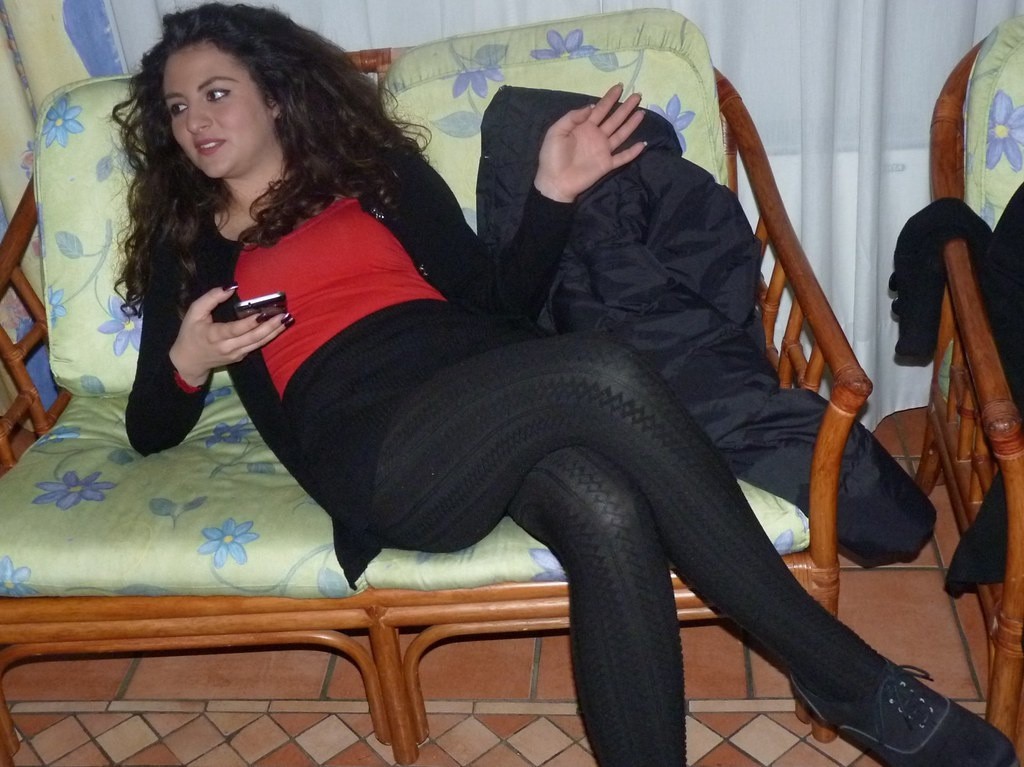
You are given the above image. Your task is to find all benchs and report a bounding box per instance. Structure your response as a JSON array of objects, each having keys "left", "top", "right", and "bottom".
[{"left": 0, "top": 46, "right": 875, "bottom": 765}]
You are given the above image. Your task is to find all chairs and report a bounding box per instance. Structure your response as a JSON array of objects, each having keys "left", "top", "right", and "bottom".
[{"left": 911, "top": 38, "right": 1024, "bottom": 765}]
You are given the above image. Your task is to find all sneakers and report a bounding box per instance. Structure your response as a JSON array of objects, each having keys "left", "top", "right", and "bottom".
[{"left": 790, "top": 653, "right": 1020, "bottom": 767}]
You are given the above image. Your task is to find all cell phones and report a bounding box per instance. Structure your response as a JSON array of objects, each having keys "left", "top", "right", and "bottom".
[{"left": 235, "top": 292, "right": 287, "bottom": 322}]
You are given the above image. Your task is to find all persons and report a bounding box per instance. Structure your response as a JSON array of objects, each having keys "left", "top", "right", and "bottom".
[{"left": 111, "top": 3, "right": 1015, "bottom": 767}]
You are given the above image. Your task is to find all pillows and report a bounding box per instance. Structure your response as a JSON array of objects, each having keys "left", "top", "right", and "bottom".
[
  {"left": 962, "top": 8, "right": 1023, "bottom": 230},
  {"left": 383, "top": 9, "right": 726, "bottom": 236},
  {"left": 34, "top": 75, "right": 229, "bottom": 398}
]
